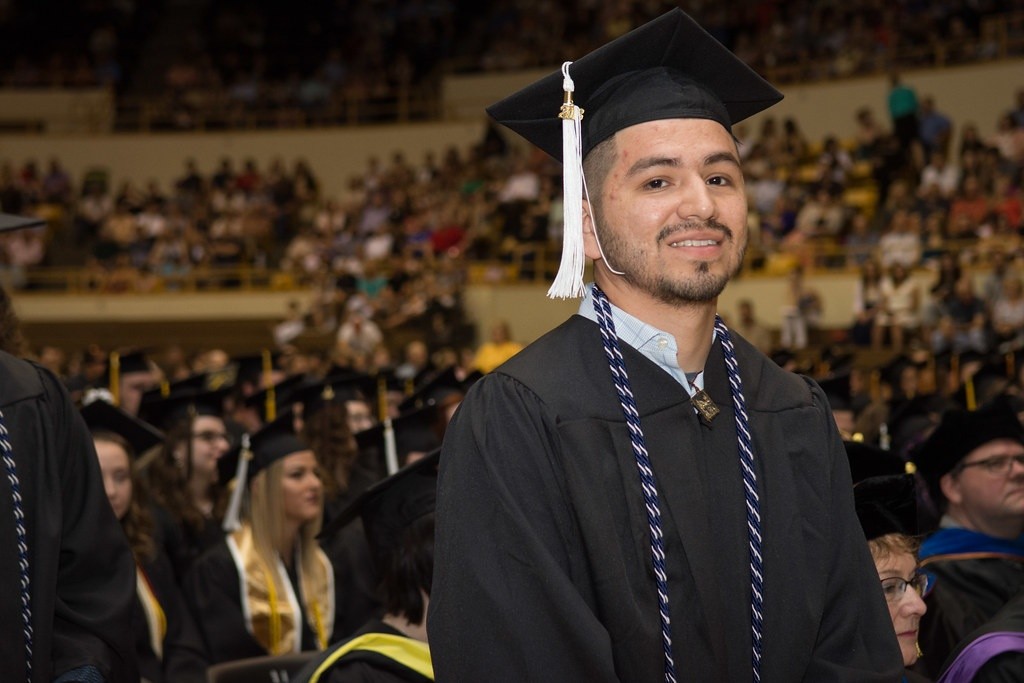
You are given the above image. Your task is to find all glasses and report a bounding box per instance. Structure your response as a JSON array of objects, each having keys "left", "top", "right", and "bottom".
[
  {"left": 880, "top": 576, "right": 919, "bottom": 605},
  {"left": 951, "top": 451, "right": 1023, "bottom": 484},
  {"left": 193, "top": 429, "right": 233, "bottom": 443}
]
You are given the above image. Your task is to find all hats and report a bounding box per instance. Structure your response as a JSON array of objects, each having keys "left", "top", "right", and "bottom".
[
  {"left": 2, "top": 208, "right": 486, "bottom": 530},
  {"left": 486, "top": 6, "right": 784, "bottom": 300},
  {"left": 768, "top": 341, "right": 1024, "bottom": 544},
  {"left": 314, "top": 444, "right": 441, "bottom": 566}
]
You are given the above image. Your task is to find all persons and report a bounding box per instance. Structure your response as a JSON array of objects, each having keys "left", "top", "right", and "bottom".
[
  {"left": 714, "top": 0, "right": 1024, "bottom": 683},
  {"left": 426, "top": 7, "right": 908, "bottom": 683},
  {"left": 0, "top": 0, "right": 716, "bottom": 683}
]
